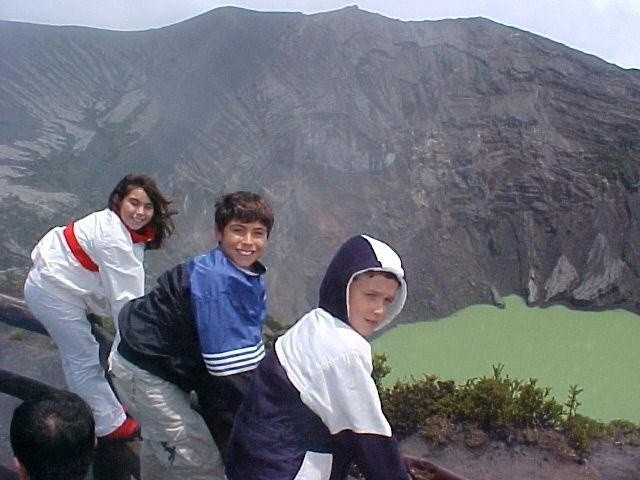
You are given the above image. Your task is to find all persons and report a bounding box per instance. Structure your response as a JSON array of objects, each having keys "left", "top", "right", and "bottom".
[
  {"left": 8, "top": 389, "right": 97, "bottom": 478},
  {"left": 20, "top": 168, "right": 176, "bottom": 443},
  {"left": 105, "top": 189, "right": 277, "bottom": 480},
  {"left": 222, "top": 231, "right": 410, "bottom": 479}
]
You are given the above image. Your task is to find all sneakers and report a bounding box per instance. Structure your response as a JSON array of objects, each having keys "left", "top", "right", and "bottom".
[{"left": 97, "top": 418, "right": 140, "bottom": 444}]
